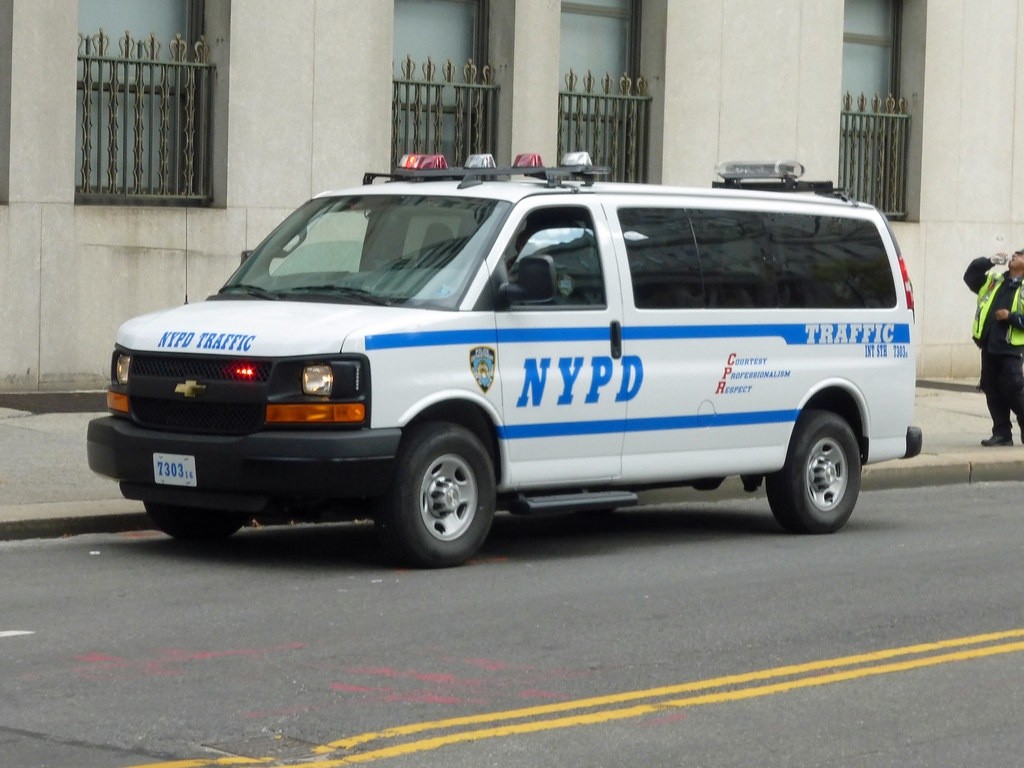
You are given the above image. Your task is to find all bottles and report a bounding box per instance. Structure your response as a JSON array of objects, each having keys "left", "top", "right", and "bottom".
[{"left": 990, "top": 255, "right": 1012, "bottom": 265}]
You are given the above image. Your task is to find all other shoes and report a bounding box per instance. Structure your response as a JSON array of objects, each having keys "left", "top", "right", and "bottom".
[{"left": 982, "top": 433, "right": 1014, "bottom": 447}]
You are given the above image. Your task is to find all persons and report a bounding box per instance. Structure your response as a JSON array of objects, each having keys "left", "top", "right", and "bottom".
[
  {"left": 963, "top": 248, "right": 1024, "bottom": 448},
  {"left": 503, "top": 227, "right": 582, "bottom": 306}
]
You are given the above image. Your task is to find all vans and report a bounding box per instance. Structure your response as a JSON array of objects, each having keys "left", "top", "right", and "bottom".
[{"left": 83, "top": 152, "right": 928, "bottom": 571}]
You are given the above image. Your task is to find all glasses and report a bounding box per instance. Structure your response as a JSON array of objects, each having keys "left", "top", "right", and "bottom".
[{"left": 1015, "top": 251, "right": 1024, "bottom": 256}]
